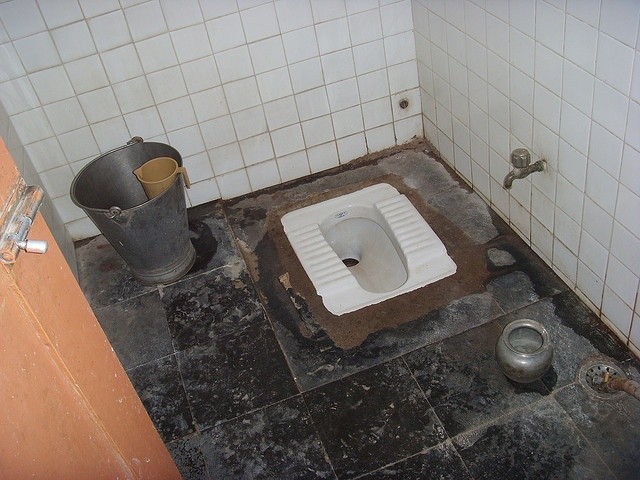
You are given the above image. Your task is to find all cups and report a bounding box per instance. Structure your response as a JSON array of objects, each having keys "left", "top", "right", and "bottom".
[{"left": 134, "top": 159, "right": 189, "bottom": 198}]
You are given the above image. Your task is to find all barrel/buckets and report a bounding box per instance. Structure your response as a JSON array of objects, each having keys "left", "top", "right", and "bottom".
[{"left": 69, "top": 137, "right": 196, "bottom": 285}]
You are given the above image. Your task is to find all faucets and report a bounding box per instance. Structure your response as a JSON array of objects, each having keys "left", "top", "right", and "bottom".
[{"left": 504, "top": 148, "right": 545, "bottom": 191}]
